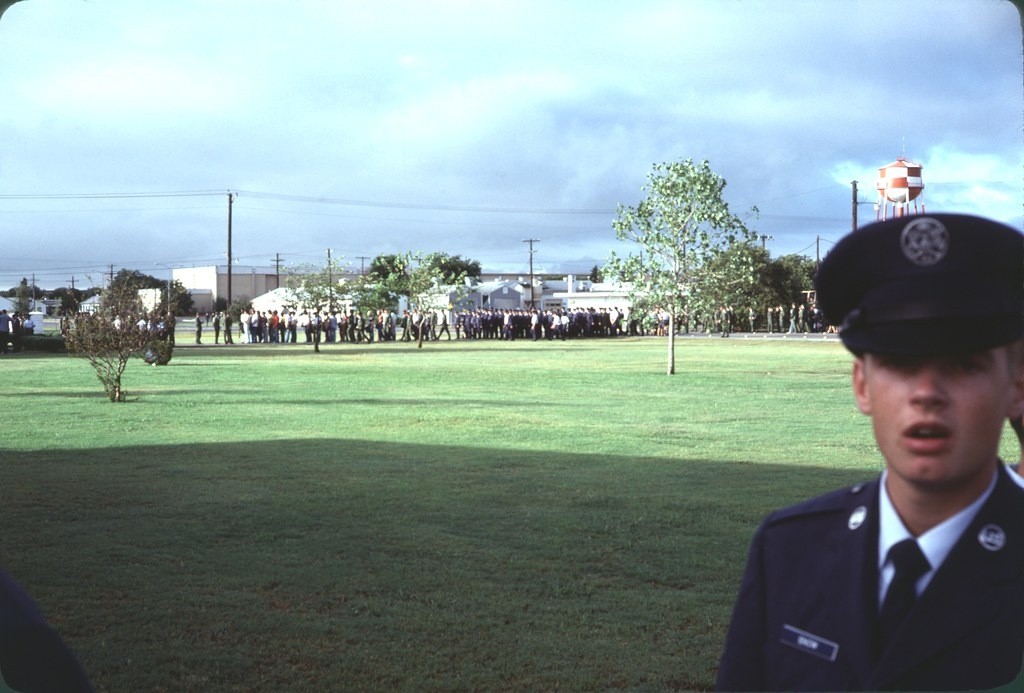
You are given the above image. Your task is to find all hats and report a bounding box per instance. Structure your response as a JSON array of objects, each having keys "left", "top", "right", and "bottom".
[{"left": 815, "top": 214, "right": 1024, "bottom": 353}]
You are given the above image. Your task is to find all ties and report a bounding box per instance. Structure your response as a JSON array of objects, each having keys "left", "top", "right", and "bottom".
[{"left": 877, "top": 539, "right": 932, "bottom": 661}]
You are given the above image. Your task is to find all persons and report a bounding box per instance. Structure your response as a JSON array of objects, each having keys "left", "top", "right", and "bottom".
[
  {"left": 717, "top": 213, "right": 1024, "bottom": 693},
  {"left": 0, "top": 305, "right": 837, "bottom": 352}
]
